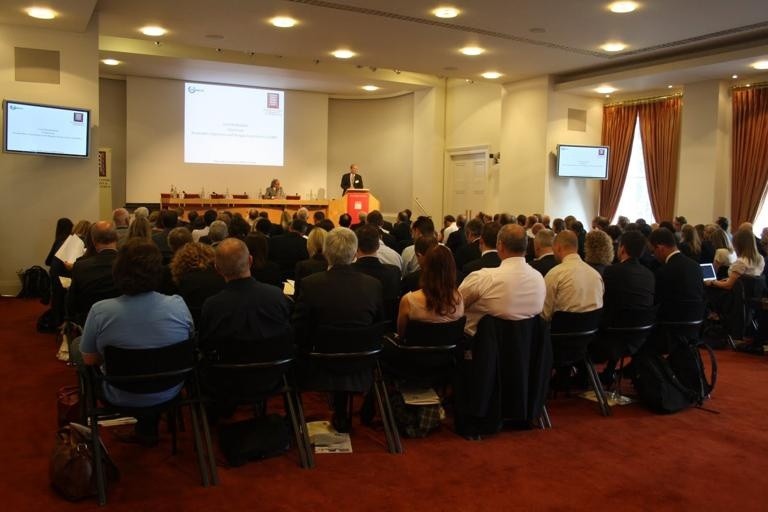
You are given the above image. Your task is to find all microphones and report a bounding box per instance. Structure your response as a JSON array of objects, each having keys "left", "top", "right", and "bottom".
[
  {"left": 212, "top": 190, "right": 219, "bottom": 196},
  {"left": 294, "top": 191, "right": 298, "bottom": 198},
  {"left": 243, "top": 191, "right": 247, "bottom": 196},
  {"left": 180, "top": 189, "right": 188, "bottom": 196}
]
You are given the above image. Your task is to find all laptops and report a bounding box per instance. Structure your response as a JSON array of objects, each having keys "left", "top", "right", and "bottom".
[{"left": 697, "top": 262, "right": 720, "bottom": 282}]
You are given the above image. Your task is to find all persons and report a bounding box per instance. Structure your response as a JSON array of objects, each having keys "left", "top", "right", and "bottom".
[
  {"left": 39, "top": 206, "right": 768, "bottom": 446},
  {"left": 340, "top": 162, "right": 363, "bottom": 197},
  {"left": 262, "top": 178, "right": 285, "bottom": 201}
]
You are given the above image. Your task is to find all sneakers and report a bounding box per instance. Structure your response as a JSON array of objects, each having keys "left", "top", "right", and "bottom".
[
  {"left": 115, "top": 428, "right": 162, "bottom": 447},
  {"left": 331, "top": 406, "right": 375, "bottom": 433},
  {"left": 40, "top": 289, "right": 51, "bottom": 305}
]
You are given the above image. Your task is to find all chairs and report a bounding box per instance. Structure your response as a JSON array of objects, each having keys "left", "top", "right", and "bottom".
[{"left": 160, "top": 192, "right": 302, "bottom": 209}]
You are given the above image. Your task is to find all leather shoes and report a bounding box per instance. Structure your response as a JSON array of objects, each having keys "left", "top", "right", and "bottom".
[{"left": 736, "top": 342, "right": 765, "bottom": 356}]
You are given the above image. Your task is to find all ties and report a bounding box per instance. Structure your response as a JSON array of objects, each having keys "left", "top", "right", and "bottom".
[{"left": 351, "top": 174, "right": 356, "bottom": 185}]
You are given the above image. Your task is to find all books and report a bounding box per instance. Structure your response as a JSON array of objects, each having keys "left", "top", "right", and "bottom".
[{"left": 314, "top": 432, "right": 354, "bottom": 454}]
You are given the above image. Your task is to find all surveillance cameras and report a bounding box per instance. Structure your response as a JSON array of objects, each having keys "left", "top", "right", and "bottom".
[{"left": 488, "top": 151, "right": 500, "bottom": 159}]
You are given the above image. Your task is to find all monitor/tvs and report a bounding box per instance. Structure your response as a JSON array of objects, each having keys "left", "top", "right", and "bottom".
[
  {"left": 3, "top": 99, "right": 91, "bottom": 159},
  {"left": 556, "top": 144, "right": 610, "bottom": 181}
]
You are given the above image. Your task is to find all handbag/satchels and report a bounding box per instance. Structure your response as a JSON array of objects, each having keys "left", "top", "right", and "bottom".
[
  {"left": 216, "top": 412, "right": 293, "bottom": 469},
  {"left": 379, "top": 390, "right": 445, "bottom": 441},
  {"left": 47, "top": 423, "right": 122, "bottom": 500},
  {"left": 55, "top": 382, "right": 87, "bottom": 428}
]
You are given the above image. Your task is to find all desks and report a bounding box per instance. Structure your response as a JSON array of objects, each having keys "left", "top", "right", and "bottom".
[{"left": 159, "top": 197, "right": 333, "bottom": 230}]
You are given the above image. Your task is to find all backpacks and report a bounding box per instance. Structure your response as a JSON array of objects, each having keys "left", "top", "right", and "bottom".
[
  {"left": 21, "top": 265, "right": 52, "bottom": 299},
  {"left": 629, "top": 331, "right": 718, "bottom": 415}
]
